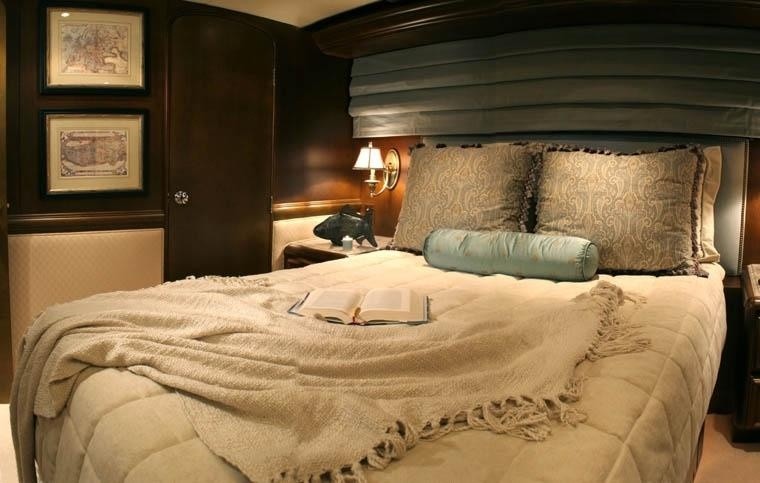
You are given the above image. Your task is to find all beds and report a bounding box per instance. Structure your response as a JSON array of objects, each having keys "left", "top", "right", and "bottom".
[{"left": 9, "top": 134, "right": 749, "bottom": 482}]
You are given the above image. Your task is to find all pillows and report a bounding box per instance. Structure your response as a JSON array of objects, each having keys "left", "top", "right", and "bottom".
[
  {"left": 423, "top": 227, "right": 599, "bottom": 280},
  {"left": 388, "top": 143, "right": 542, "bottom": 256},
  {"left": 697, "top": 144, "right": 723, "bottom": 265},
  {"left": 534, "top": 143, "right": 709, "bottom": 278}
]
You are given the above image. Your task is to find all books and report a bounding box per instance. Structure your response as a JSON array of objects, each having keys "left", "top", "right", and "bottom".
[{"left": 287, "top": 287, "right": 432, "bottom": 328}]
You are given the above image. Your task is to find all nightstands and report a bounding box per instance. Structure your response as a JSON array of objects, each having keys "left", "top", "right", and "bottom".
[
  {"left": 284, "top": 235, "right": 394, "bottom": 268},
  {"left": 732, "top": 263, "right": 759, "bottom": 445}
]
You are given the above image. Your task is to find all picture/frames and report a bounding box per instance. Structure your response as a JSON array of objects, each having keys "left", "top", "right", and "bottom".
[
  {"left": 38, "top": 1, "right": 151, "bottom": 97},
  {"left": 43, "top": 109, "right": 150, "bottom": 197}
]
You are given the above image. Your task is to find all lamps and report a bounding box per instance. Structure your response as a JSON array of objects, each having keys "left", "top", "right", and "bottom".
[{"left": 353, "top": 140, "right": 400, "bottom": 199}]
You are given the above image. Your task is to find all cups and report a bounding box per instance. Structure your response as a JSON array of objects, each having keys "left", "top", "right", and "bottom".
[{"left": 341, "top": 239, "right": 353, "bottom": 251}]
